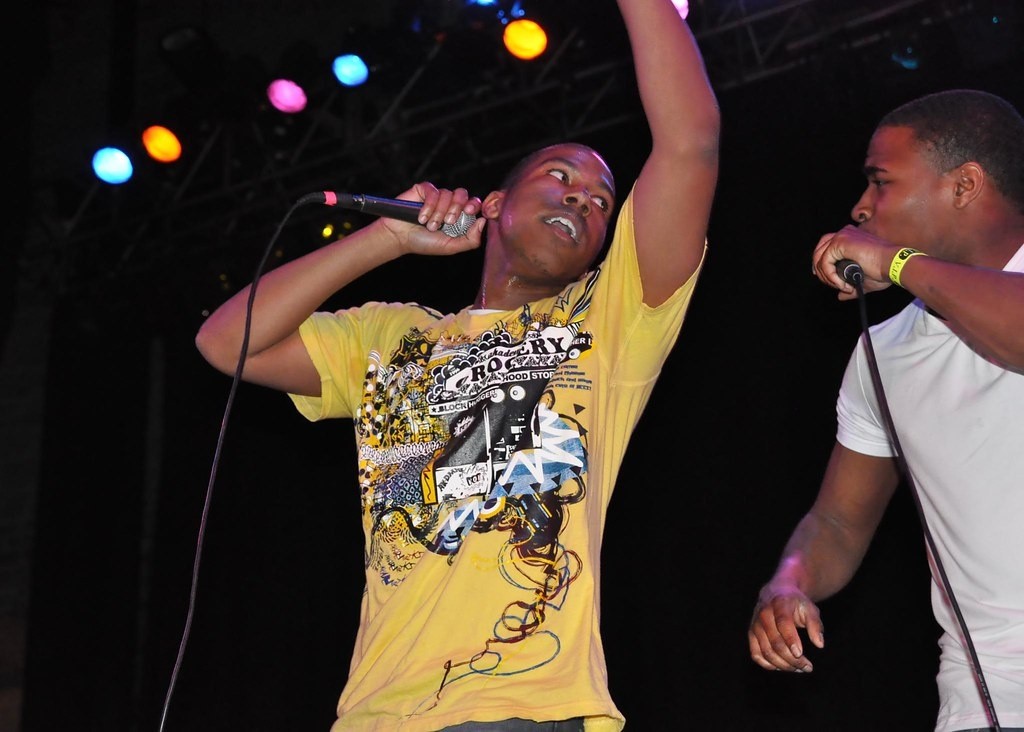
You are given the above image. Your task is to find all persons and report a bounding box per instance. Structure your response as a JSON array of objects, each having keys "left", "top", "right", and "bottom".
[
  {"left": 193, "top": 2, "right": 735, "bottom": 731},
  {"left": 744, "top": 82, "right": 1024, "bottom": 732}
]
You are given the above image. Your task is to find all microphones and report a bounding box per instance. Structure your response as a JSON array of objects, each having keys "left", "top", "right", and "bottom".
[
  {"left": 298, "top": 191, "right": 478, "bottom": 239},
  {"left": 835, "top": 258, "right": 863, "bottom": 285}
]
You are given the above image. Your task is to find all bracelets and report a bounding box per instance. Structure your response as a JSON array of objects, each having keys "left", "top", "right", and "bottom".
[{"left": 888, "top": 244, "right": 925, "bottom": 290}]
